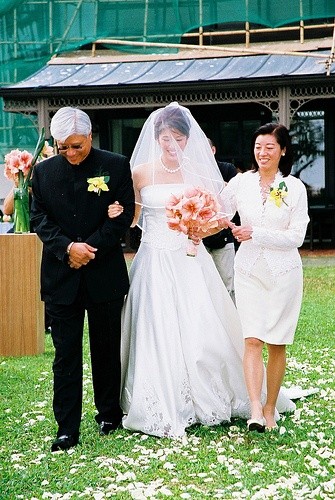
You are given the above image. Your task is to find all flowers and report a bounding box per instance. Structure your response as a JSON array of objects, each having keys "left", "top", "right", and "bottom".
[
  {"left": 3, "top": 126, "right": 54, "bottom": 231},
  {"left": 165, "top": 187, "right": 220, "bottom": 256},
  {"left": 87, "top": 172, "right": 110, "bottom": 196},
  {"left": 269, "top": 181, "right": 289, "bottom": 208}
]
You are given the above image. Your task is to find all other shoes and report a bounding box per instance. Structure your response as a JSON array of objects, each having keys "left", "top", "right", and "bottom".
[
  {"left": 99, "top": 419, "right": 121, "bottom": 437},
  {"left": 51, "top": 433, "right": 79, "bottom": 453}
]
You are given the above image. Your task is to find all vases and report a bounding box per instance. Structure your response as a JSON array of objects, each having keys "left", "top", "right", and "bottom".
[{"left": 11, "top": 184, "right": 30, "bottom": 235}]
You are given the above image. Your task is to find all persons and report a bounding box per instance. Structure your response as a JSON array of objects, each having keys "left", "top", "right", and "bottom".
[
  {"left": 108, "top": 101, "right": 308, "bottom": 441},
  {"left": 0, "top": 108, "right": 137, "bottom": 453}
]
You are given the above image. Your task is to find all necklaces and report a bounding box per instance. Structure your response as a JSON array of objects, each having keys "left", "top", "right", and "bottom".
[{"left": 160, "top": 152, "right": 186, "bottom": 172}]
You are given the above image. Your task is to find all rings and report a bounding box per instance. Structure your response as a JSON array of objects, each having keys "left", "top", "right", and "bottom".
[{"left": 236, "top": 236, "right": 239, "bottom": 239}]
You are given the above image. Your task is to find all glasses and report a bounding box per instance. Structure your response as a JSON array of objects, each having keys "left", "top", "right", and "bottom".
[{"left": 56, "top": 134, "right": 89, "bottom": 150}]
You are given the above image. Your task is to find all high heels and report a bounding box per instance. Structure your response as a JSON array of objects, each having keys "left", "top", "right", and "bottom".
[
  {"left": 246, "top": 415, "right": 266, "bottom": 434},
  {"left": 263, "top": 407, "right": 278, "bottom": 433}
]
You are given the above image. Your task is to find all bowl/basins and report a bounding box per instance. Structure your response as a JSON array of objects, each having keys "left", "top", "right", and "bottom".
[{"left": 0, "top": 222, "right": 14, "bottom": 234}]
